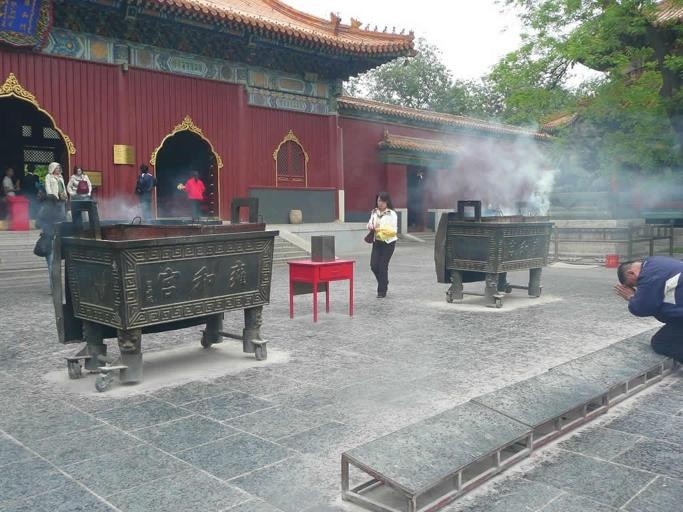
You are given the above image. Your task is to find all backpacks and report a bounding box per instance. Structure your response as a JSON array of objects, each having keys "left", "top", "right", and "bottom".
[{"left": 76, "top": 180, "right": 89, "bottom": 194}]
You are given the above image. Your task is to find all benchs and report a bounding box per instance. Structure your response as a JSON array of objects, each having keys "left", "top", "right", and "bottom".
[{"left": 552, "top": 224, "right": 676, "bottom": 262}]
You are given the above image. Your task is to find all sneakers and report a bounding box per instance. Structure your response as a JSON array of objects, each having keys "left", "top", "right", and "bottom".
[
  {"left": 47, "top": 288, "right": 53, "bottom": 295},
  {"left": 377, "top": 287, "right": 388, "bottom": 298}
]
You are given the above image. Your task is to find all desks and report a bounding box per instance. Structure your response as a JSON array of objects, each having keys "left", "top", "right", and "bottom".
[{"left": 287, "top": 259, "right": 355, "bottom": 323}]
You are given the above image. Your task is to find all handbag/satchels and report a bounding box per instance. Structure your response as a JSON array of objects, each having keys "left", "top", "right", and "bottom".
[
  {"left": 364, "top": 229, "right": 374, "bottom": 243},
  {"left": 200, "top": 193, "right": 208, "bottom": 202},
  {"left": 59, "top": 192, "right": 68, "bottom": 200},
  {"left": 36, "top": 190, "right": 44, "bottom": 199},
  {"left": 33, "top": 232, "right": 51, "bottom": 257},
  {"left": 134, "top": 185, "right": 144, "bottom": 195}
]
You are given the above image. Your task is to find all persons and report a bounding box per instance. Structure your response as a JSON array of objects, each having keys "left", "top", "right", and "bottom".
[
  {"left": 1, "top": 166, "right": 19, "bottom": 196},
  {"left": 612, "top": 255, "right": 683, "bottom": 362},
  {"left": 19, "top": 164, "right": 40, "bottom": 219},
  {"left": 363, "top": 190, "right": 399, "bottom": 299},
  {"left": 44, "top": 162, "right": 67, "bottom": 214},
  {"left": 65, "top": 166, "right": 92, "bottom": 222},
  {"left": 176, "top": 170, "right": 205, "bottom": 217},
  {"left": 133, "top": 163, "right": 157, "bottom": 218}
]
